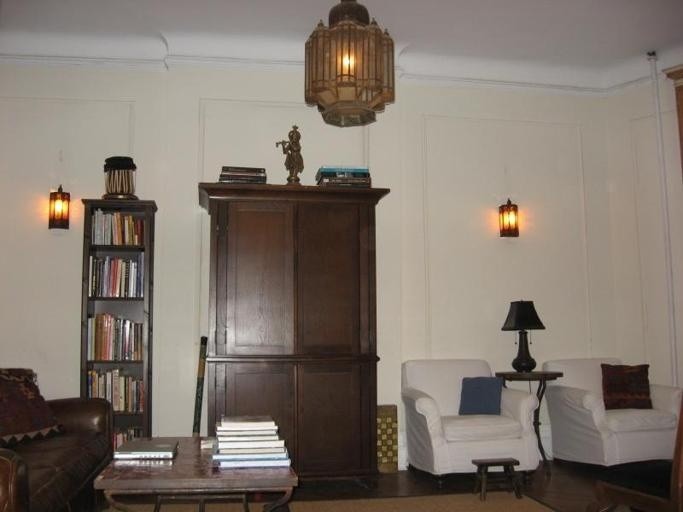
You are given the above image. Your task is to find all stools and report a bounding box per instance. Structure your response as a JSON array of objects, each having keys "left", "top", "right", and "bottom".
[{"left": 472, "top": 458, "right": 521, "bottom": 501}]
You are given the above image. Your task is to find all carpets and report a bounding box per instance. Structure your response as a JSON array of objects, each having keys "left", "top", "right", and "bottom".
[{"left": 100, "top": 491, "right": 561, "bottom": 512}]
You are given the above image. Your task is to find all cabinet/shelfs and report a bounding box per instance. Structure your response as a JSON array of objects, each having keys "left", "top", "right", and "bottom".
[
  {"left": 80, "top": 197, "right": 158, "bottom": 449},
  {"left": 198, "top": 181, "right": 391, "bottom": 487}
]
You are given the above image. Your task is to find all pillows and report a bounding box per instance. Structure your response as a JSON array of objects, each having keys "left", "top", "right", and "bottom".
[
  {"left": 601, "top": 362, "right": 653, "bottom": 410},
  {"left": 1, "top": 372, "right": 66, "bottom": 448},
  {"left": 458, "top": 375, "right": 504, "bottom": 415}
]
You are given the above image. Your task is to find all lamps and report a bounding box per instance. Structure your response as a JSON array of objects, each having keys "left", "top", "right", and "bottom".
[
  {"left": 499, "top": 198, "right": 519, "bottom": 237},
  {"left": 48, "top": 184, "right": 71, "bottom": 230},
  {"left": 501, "top": 301, "right": 545, "bottom": 373},
  {"left": 305, "top": 1, "right": 396, "bottom": 127}
]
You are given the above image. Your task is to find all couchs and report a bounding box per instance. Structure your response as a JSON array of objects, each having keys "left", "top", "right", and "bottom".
[
  {"left": 402, "top": 360, "right": 540, "bottom": 488},
  {"left": 0, "top": 368, "right": 115, "bottom": 512},
  {"left": 545, "top": 357, "right": 681, "bottom": 466}
]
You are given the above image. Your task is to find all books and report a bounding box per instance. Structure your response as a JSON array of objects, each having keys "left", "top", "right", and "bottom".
[
  {"left": 85, "top": 368, "right": 147, "bottom": 413},
  {"left": 114, "top": 436, "right": 179, "bottom": 460},
  {"left": 115, "top": 424, "right": 148, "bottom": 444},
  {"left": 88, "top": 253, "right": 148, "bottom": 299},
  {"left": 212, "top": 411, "right": 291, "bottom": 468},
  {"left": 218, "top": 166, "right": 266, "bottom": 184},
  {"left": 88, "top": 205, "right": 149, "bottom": 246},
  {"left": 315, "top": 166, "right": 370, "bottom": 187},
  {"left": 86, "top": 314, "right": 142, "bottom": 362}
]
